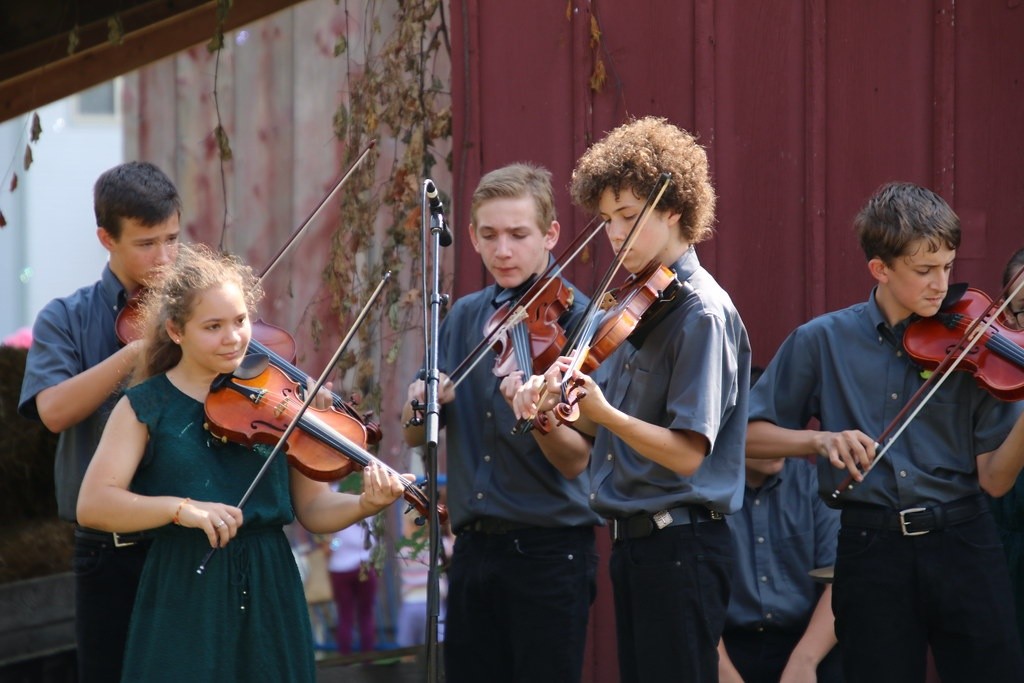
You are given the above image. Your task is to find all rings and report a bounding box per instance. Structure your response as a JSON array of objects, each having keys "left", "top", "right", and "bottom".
[
  {"left": 215, "top": 521, "right": 225, "bottom": 530},
  {"left": 224, "top": 515, "right": 231, "bottom": 522}
]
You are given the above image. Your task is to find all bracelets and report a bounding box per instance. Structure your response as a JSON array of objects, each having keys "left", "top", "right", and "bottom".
[{"left": 174, "top": 497, "right": 191, "bottom": 526}]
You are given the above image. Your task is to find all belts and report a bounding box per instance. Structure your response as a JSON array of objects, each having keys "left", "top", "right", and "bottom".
[
  {"left": 74, "top": 527, "right": 158, "bottom": 547},
  {"left": 614, "top": 503, "right": 724, "bottom": 541},
  {"left": 840, "top": 491, "right": 1004, "bottom": 535},
  {"left": 463, "top": 517, "right": 536, "bottom": 535}
]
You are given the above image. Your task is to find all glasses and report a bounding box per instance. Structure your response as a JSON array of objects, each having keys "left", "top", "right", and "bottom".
[{"left": 1009, "top": 305, "right": 1024, "bottom": 328}]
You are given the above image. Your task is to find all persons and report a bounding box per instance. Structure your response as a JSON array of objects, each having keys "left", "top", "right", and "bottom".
[
  {"left": 717, "top": 183, "right": 1024, "bottom": 683},
  {"left": 17, "top": 161, "right": 416, "bottom": 683},
  {"left": 512, "top": 115, "right": 753, "bottom": 683},
  {"left": 401, "top": 164, "right": 607, "bottom": 683}
]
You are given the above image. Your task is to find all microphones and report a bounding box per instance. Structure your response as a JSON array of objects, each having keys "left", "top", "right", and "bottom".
[{"left": 424, "top": 179, "right": 453, "bottom": 247}]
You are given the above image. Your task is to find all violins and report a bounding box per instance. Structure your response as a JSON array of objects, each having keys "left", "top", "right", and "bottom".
[
  {"left": 901, "top": 282, "right": 1024, "bottom": 402},
  {"left": 202, "top": 352, "right": 449, "bottom": 527},
  {"left": 482, "top": 275, "right": 575, "bottom": 437},
  {"left": 552, "top": 259, "right": 682, "bottom": 427},
  {"left": 113, "top": 284, "right": 384, "bottom": 446}
]
